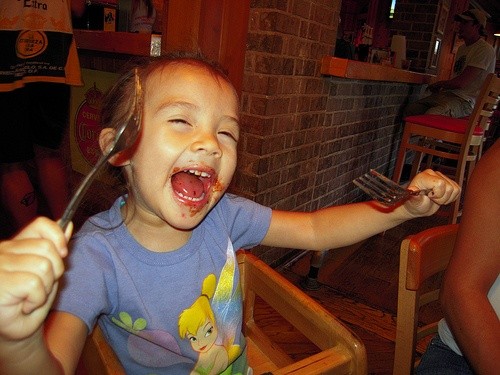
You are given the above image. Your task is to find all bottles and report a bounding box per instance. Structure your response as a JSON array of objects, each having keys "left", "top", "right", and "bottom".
[
  {"left": 40, "top": 157, "right": 72, "bottom": 218},
  {"left": 1, "top": 170, "right": 39, "bottom": 223},
  {"left": 89, "top": 0, "right": 119, "bottom": 32}
]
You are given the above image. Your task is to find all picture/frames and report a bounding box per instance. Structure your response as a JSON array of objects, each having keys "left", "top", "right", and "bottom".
[
  {"left": 436, "top": 4, "right": 449, "bottom": 35},
  {"left": 429, "top": 37, "right": 442, "bottom": 70}
]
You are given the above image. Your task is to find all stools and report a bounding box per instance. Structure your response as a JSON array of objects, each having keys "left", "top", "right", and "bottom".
[{"left": 381, "top": 74, "right": 500, "bottom": 236}]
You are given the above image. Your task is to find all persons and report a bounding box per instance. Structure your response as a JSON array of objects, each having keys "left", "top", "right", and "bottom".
[
  {"left": 0, "top": 51, "right": 462, "bottom": 375},
  {"left": 410, "top": 9, "right": 496, "bottom": 118},
  {"left": 416, "top": 138, "right": 500, "bottom": 375},
  {"left": 0, "top": 0, "right": 84, "bottom": 234}
]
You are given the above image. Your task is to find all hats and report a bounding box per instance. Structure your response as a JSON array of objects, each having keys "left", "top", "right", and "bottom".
[{"left": 455, "top": 9, "right": 487, "bottom": 28}]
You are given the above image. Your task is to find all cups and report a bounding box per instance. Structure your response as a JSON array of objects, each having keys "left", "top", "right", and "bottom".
[{"left": 402, "top": 59, "right": 412, "bottom": 70}]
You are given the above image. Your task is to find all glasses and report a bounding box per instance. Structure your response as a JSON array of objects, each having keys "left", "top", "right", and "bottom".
[{"left": 463, "top": 11, "right": 475, "bottom": 18}]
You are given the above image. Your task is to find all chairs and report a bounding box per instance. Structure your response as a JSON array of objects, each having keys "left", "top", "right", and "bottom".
[{"left": 393, "top": 224, "right": 460, "bottom": 375}]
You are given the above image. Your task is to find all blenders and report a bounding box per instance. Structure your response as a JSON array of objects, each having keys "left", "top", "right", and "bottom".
[{"left": 383, "top": 35, "right": 405, "bottom": 67}]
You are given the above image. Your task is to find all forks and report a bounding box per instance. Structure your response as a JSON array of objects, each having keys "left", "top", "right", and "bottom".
[
  {"left": 352, "top": 169, "right": 431, "bottom": 206},
  {"left": 58, "top": 67, "right": 143, "bottom": 234}
]
